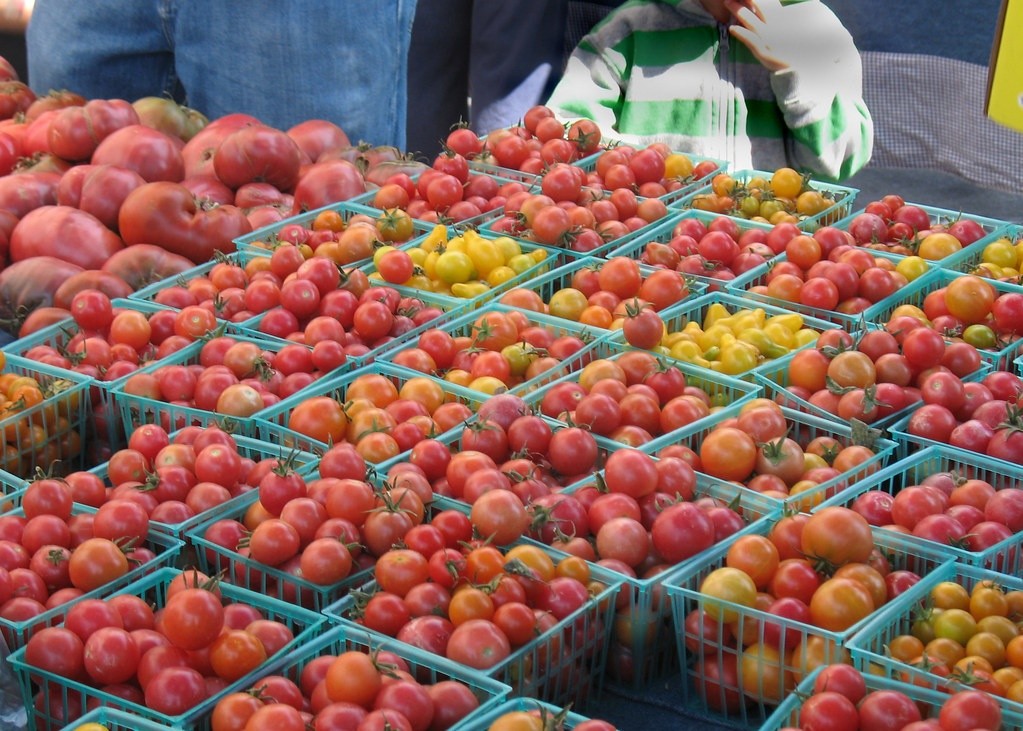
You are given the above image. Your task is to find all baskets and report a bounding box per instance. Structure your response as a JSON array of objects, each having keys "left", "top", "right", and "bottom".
[{"left": 0, "top": 122, "right": 1023, "bottom": 730}]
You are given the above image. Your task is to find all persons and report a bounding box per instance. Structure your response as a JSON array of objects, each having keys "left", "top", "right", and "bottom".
[
  {"left": 544, "top": 0, "right": 873, "bottom": 187},
  {"left": 25, "top": 0, "right": 416, "bottom": 163}
]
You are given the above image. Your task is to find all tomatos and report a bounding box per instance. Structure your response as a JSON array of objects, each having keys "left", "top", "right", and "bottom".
[{"left": 0, "top": 54, "right": 1023, "bottom": 731}]
